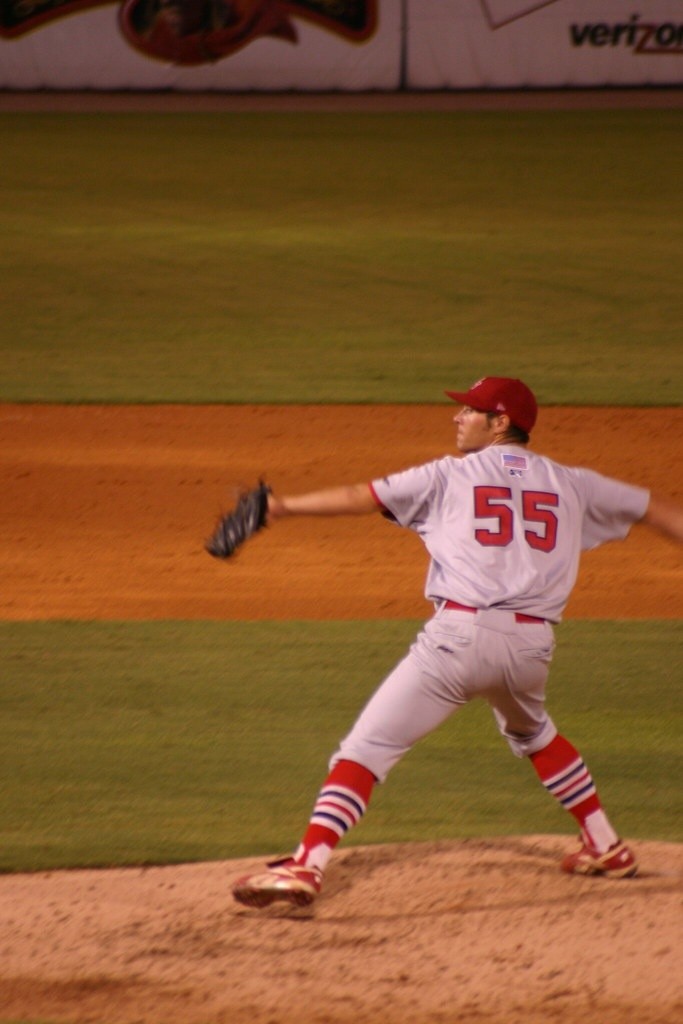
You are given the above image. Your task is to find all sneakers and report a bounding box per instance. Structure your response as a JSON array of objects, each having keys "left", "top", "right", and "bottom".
[
  {"left": 231, "top": 856, "right": 324, "bottom": 908},
  {"left": 560, "top": 831, "right": 639, "bottom": 878}
]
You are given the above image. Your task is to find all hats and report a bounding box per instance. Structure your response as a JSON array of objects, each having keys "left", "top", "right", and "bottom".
[{"left": 444, "top": 377, "right": 537, "bottom": 433}]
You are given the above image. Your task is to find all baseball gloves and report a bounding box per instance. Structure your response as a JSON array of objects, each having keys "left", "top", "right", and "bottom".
[{"left": 202, "top": 478, "right": 274, "bottom": 565}]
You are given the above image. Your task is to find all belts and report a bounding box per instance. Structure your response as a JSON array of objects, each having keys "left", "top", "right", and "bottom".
[{"left": 444, "top": 600, "right": 545, "bottom": 624}]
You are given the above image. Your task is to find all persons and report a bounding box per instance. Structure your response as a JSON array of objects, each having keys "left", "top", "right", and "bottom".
[{"left": 231, "top": 377, "right": 682, "bottom": 908}]
